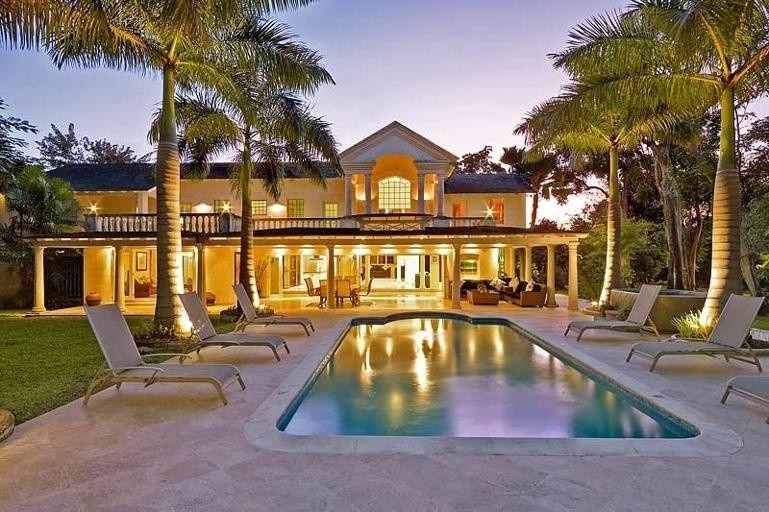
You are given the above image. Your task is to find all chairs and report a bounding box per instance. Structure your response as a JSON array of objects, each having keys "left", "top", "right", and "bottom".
[
  {"left": 626, "top": 293, "right": 766, "bottom": 372},
  {"left": 134, "top": 278, "right": 151, "bottom": 297},
  {"left": 303, "top": 274, "right": 374, "bottom": 308},
  {"left": 720, "top": 375, "right": 769, "bottom": 424},
  {"left": 176, "top": 291, "right": 289, "bottom": 361},
  {"left": 564, "top": 284, "right": 663, "bottom": 342},
  {"left": 232, "top": 283, "right": 314, "bottom": 336},
  {"left": 82, "top": 302, "right": 246, "bottom": 406}
]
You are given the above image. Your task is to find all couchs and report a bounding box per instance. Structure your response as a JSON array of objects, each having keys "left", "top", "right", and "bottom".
[{"left": 450, "top": 277, "right": 547, "bottom": 307}]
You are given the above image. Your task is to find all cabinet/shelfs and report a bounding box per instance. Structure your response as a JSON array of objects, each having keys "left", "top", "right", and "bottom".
[{"left": 460, "top": 260, "right": 478, "bottom": 275}]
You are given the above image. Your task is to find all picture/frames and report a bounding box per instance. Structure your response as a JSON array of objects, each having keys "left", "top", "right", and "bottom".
[
  {"left": 136, "top": 251, "right": 147, "bottom": 271},
  {"left": 234, "top": 251, "right": 241, "bottom": 285}
]
